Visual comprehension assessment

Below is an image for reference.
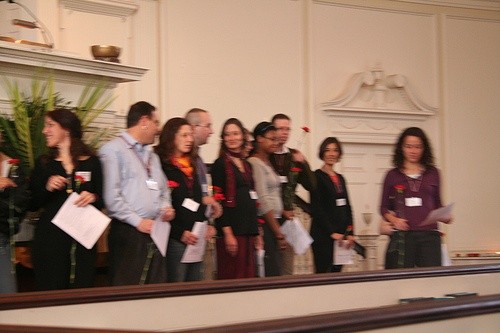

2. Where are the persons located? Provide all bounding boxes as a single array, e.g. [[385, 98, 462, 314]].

[[211, 118, 260, 280], [227, 128, 265, 276], [308, 136, 356, 274], [22, 109, 103, 287], [380, 128, 454, 269], [246, 120, 293, 277], [0, 127, 39, 294], [265, 113, 317, 275], [153, 118, 206, 283], [184, 108, 223, 225], [94, 101, 176, 284]]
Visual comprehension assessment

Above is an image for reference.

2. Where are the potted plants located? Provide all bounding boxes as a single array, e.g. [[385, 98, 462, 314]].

[[0, 71, 122, 293]]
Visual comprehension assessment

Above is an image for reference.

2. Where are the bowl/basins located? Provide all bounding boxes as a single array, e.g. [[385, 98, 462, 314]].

[[91, 45, 120, 63]]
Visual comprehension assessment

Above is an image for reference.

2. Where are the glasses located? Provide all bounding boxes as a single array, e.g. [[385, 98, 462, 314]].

[[261, 134, 278, 141], [195, 123, 211, 128]]
